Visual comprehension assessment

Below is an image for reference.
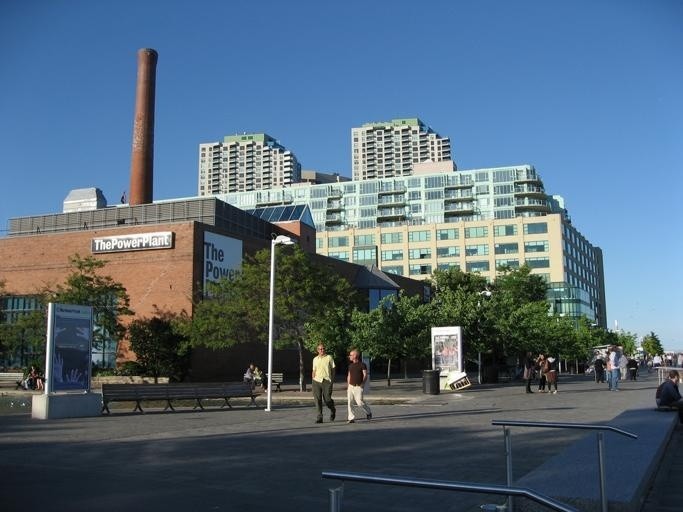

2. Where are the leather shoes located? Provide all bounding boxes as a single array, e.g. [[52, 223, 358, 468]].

[[314, 420, 322, 424], [329, 413, 334, 421]]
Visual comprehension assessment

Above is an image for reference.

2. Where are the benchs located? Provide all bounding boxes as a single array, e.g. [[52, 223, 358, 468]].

[[101, 381, 262, 415], [265, 373, 284, 392]]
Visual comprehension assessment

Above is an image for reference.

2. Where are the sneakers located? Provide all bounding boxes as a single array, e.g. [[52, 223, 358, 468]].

[[526, 390, 557, 394], [346, 420, 354, 423], [366, 413, 370, 420]]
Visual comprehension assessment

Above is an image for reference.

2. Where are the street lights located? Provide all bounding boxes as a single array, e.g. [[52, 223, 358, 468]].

[[477, 286, 491, 384], [264, 232, 295, 412]]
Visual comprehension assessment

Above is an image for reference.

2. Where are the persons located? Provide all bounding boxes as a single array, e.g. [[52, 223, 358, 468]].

[[244, 362, 256, 385], [543, 353, 560, 395], [656, 370, 682, 424], [32, 369, 36, 379], [347, 349, 372, 424], [23, 365, 38, 390], [254, 364, 267, 394], [594, 346, 683, 395], [522, 351, 537, 394], [536, 354, 547, 394], [310, 344, 338, 423], [35, 367, 45, 392]]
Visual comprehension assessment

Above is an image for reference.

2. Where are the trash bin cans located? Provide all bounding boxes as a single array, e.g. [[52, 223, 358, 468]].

[[422, 369, 441, 395]]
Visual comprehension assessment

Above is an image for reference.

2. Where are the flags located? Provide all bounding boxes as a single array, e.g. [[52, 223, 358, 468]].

[[120, 192, 126, 205]]
